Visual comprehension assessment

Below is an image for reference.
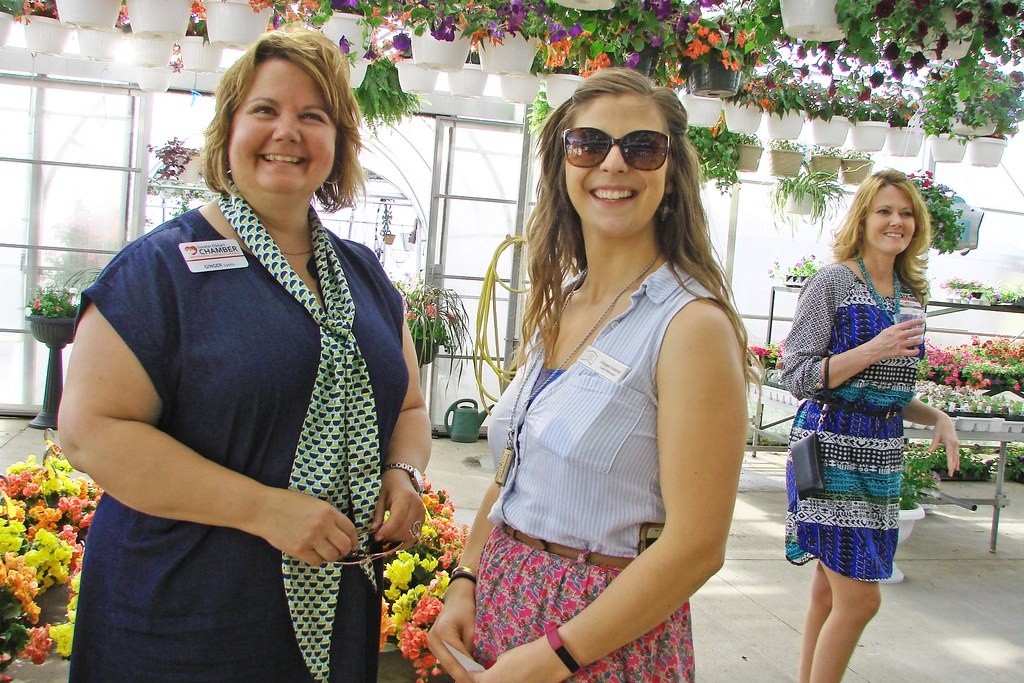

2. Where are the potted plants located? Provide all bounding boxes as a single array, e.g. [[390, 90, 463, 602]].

[[380, 202, 396, 246], [767, 170, 853, 244]]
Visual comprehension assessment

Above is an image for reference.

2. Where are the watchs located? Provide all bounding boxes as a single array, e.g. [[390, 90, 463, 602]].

[[381, 462, 424, 492]]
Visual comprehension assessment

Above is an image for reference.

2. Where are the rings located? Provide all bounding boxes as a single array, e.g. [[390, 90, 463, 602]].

[[409, 520, 424, 539]]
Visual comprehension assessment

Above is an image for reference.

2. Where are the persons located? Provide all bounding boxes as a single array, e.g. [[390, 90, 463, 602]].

[[58, 28, 434, 683], [782, 168, 960, 683], [426, 67, 766, 683]]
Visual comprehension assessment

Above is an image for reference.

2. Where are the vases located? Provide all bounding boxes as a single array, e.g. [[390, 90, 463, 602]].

[[21, 314, 81, 430], [749, 357, 1024, 432], [414, 334, 443, 363], [0, 0, 274, 95], [786, 275, 1024, 306], [879, 465, 1024, 584], [320, 0, 1024, 249]]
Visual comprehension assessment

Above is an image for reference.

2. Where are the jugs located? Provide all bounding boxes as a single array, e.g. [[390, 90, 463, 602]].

[[444, 398, 494, 442]]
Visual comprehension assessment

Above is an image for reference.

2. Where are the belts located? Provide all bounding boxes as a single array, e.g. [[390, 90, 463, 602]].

[[501, 522, 634, 570]]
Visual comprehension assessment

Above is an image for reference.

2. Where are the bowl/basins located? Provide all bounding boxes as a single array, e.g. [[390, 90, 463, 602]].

[[391, 249, 409, 263]]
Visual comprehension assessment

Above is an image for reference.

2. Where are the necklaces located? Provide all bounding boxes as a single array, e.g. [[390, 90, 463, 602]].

[[494, 249, 663, 488], [853, 253, 901, 322], [283, 250, 313, 255]]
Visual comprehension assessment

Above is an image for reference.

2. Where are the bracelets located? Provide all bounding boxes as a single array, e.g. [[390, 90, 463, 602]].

[[545, 623, 583, 678], [452, 566, 477, 576], [447, 573, 478, 586]]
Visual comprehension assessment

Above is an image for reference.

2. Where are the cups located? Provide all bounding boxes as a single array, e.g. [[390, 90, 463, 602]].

[[893, 313, 926, 344], [400, 233, 414, 251]]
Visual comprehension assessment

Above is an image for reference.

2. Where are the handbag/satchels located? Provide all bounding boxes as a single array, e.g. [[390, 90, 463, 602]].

[[792, 357, 837, 502]]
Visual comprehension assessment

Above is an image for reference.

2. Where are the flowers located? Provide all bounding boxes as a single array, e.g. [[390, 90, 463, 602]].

[[906, 169, 966, 257], [14, 0, 1024, 160], [0, 445, 471, 683], [749, 337, 1023, 414], [28, 285, 82, 319], [767, 257, 1020, 296], [898, 442, 1024, 510], [394, 281, 473, 345]]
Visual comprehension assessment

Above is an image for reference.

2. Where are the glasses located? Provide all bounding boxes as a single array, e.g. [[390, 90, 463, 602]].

[[334, 528, 404, 565], [560, 126, 674, 171]]
